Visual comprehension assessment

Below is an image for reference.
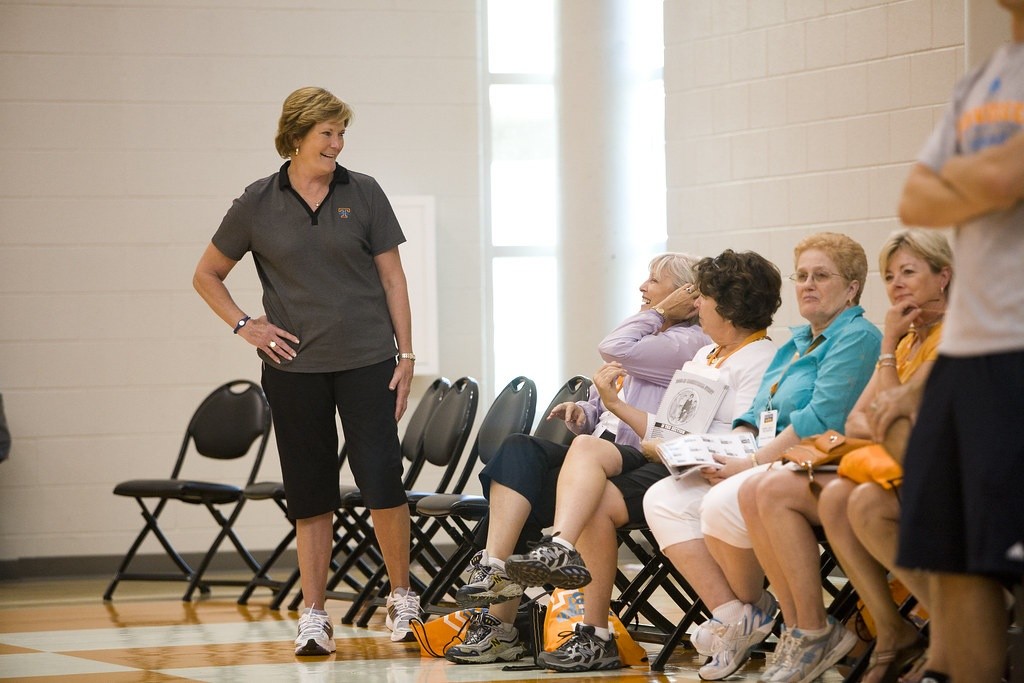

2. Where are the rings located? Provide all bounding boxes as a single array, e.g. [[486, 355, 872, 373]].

[[269, 342, 276, 348], [686, 288, 693, 294], [871, 402, 877, 409]]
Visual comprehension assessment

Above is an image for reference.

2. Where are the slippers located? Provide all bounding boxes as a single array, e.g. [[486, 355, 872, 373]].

[[861, 632, 928, 682]]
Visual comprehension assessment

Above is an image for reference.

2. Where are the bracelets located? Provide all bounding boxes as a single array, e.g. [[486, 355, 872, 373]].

[[877, 354, 897, 363], [750, 454, 758, 467], [397, 353, 416, 361], [878, 363, 897, 370]]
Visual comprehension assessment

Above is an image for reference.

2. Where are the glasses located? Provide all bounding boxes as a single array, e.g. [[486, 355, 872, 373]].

[[789, 269, 853, 286], [903, 298, 945, 333]]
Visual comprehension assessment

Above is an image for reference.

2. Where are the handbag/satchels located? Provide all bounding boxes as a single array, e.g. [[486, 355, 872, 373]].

[[767, 428, 873, 482], [837, 443, 907, 491], [409, 607, 490, 658], [542, 583, 651, 666]]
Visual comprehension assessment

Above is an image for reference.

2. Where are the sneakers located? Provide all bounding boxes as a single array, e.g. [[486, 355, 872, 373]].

[[536, 623, 621, 672], [698, 603, 777, 681], [445, 611, 528, 664], [455, 548, 524, 610], [385, 586, 425, 642], [690, 590, 778, 657], [756, 613, 858, 683], [295, 602, 337, 656], [504, 532, 593, 590]]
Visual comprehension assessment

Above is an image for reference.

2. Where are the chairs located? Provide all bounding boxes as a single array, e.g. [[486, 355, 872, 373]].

[[102, 378, 1024, 683]]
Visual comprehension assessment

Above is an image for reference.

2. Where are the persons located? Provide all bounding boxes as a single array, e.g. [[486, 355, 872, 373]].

[[193, 87, 425, 656], [894, 0, 1024, 683], [739, 228, 958, 683], [505, 248, 782, 671], [444, 252, 713, 665], [643, 233, 883, 680]]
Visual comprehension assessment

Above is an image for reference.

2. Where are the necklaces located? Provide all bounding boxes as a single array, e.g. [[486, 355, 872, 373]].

[[290, 173, 330, 207], [912, 314, 944, 331]]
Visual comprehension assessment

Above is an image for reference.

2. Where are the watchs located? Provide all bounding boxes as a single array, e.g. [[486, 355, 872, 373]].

[[233, 316, 251, 334], [651, 306, 668, 323]]
